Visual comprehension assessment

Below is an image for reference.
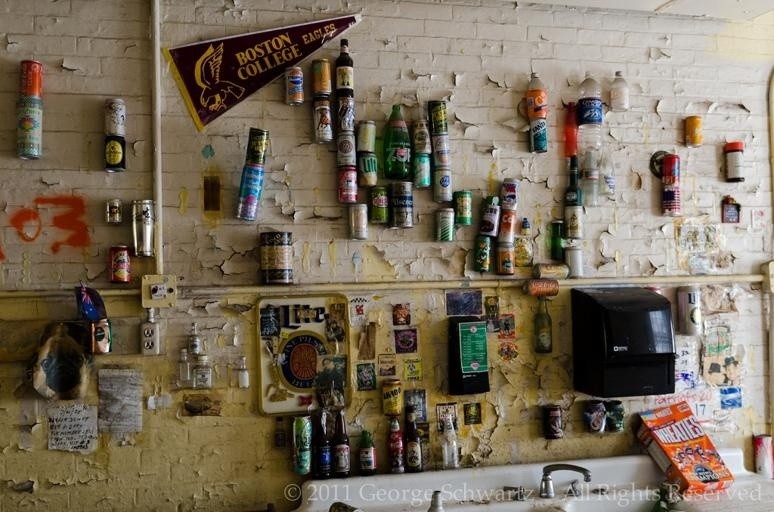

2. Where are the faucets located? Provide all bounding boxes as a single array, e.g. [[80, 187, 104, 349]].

[[539, 462, 594, 499]]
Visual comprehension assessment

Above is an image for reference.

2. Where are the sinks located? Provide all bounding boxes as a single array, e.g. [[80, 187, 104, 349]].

[[293, 448, 774, 511]]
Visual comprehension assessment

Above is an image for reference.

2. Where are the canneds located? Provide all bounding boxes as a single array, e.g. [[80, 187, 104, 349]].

[[530, 118, 547, 154], [91, 317, 112, 355], [677, 285, 701, 337], [101, 97, 126, 173], [290, 414, 313, 475], [281, 58, 518, 275], [105, 197, 156, 281], [522, 263, 571, 297], [381, 378, 402, 416], [234, 127, 294, 284], [14, 60, 43, 160], [754, 433, 773, 480], [542, 400, 625, 439], [660, 114, 704, 217]]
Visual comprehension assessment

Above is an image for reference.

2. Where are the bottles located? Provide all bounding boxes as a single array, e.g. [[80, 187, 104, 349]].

[[385, 416, 403, 473], [404, 403, 422, 470], [582, 149, 600, 207], [577, 70, 601, 150], [550, 221, 564, 258], [180, 323, 249, 389], [272, 415, 285, 448], [610, 71, 629, 113], [358, 431, 375, 475], [535, 296, 553, 352], [335, 35, 353, 96], [564, 156, 583, 238], [563, 102, 578, 157], [329, 408, 351, 477], [441, 414, 460, 467], [724, 142, 745, 182], [312, 410, 330, 480], [385, 102, 412, 177], [527, 71, 546, 119]]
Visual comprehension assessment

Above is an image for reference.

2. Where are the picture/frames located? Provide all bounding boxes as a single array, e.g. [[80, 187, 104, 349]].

[[256, 294, 352, 416]]
